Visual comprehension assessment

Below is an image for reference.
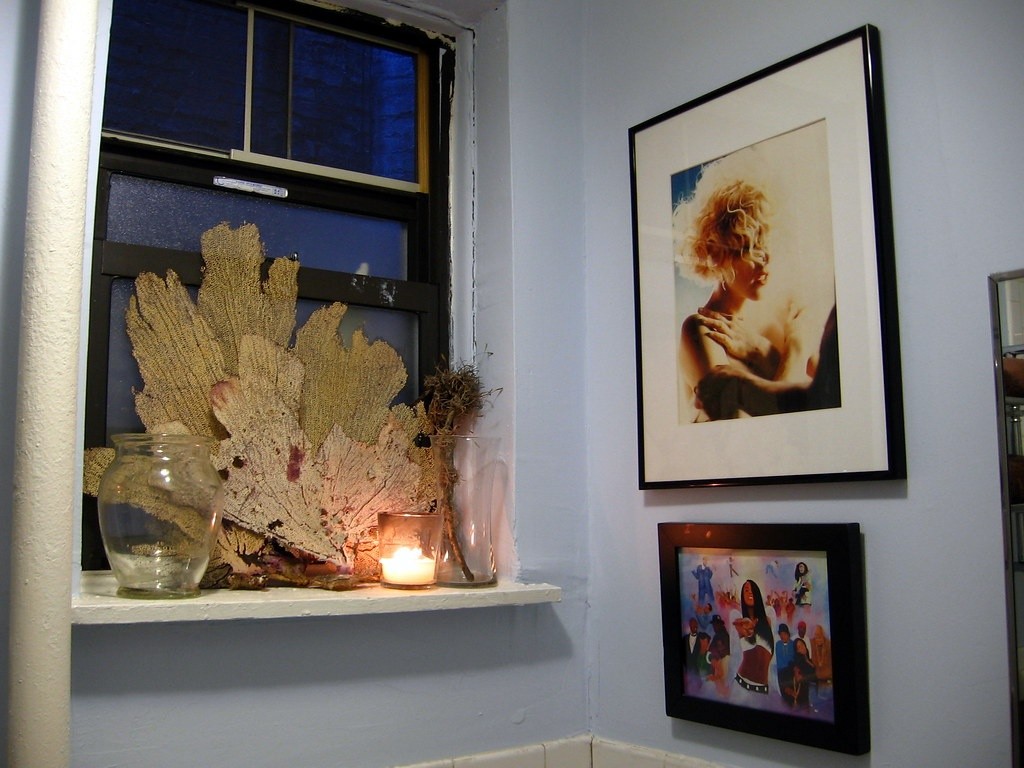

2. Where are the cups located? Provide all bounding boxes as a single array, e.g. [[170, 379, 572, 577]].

[[98, 433, 224, 598]]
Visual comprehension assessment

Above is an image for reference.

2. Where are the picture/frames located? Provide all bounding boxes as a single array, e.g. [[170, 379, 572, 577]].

[[655, 521, 871, 755], [626, 24, 908, 491]]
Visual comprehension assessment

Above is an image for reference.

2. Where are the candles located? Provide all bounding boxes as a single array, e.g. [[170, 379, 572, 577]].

[[377, 546, 434, 585]]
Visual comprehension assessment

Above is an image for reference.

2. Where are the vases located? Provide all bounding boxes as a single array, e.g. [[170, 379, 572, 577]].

[[430, 436, 498, 588], [97, 433, 224, 599]]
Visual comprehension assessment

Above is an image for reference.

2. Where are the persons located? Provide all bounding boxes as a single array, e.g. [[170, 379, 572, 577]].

[[677, 158, 842, 424]]
